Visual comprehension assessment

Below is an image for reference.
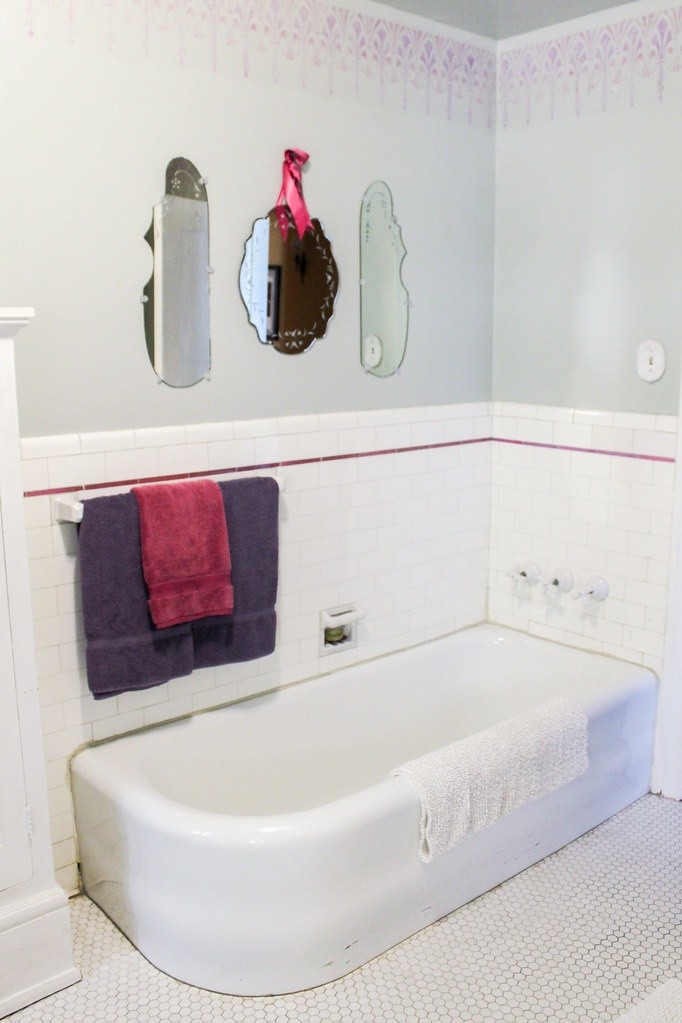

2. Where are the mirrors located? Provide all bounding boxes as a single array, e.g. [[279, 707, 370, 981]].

[[358, 179, 409, 378], [143, 157, 211, 387], [239, 204, 339, 354]]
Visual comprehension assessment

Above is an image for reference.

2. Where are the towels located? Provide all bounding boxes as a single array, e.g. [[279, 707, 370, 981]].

[[384, 697, 589, 863], [131, 480, 233, 629], [78, 477, 279, 700]]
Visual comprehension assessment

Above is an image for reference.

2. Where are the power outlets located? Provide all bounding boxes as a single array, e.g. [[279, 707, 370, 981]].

[[362, 335, 384, 368], [636, 340, 665, 383]]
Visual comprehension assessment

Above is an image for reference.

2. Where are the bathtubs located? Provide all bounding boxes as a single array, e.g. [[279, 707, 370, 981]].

[[71, 621, 660, 997]]
